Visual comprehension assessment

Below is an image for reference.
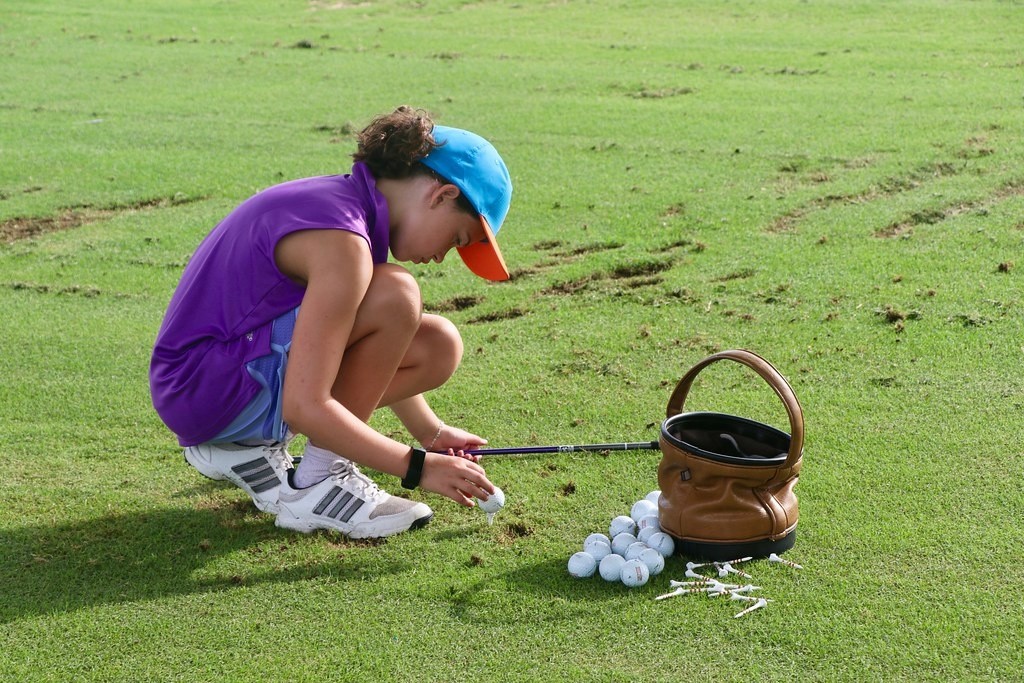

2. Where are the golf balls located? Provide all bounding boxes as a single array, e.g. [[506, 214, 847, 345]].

[[476, 485, 506, 514], [567, 489, 675, 589]]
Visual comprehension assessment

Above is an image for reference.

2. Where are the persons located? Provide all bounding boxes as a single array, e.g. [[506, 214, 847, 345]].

[[147, 106, 510, 539]]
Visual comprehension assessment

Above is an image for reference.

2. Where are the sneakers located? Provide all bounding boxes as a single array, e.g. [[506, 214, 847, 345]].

[[273, 461, 434, 538], [185, 439, 295, 516]]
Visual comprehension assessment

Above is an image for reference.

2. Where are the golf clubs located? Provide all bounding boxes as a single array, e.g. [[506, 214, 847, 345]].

[[416, 440, 662, 458]]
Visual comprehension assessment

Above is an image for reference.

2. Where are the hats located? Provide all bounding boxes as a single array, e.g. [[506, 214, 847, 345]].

[[418, 124, 512, 282]]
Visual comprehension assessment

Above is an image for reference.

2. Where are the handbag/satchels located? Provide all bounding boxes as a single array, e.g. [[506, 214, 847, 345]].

[[657, 349, 806, 560]]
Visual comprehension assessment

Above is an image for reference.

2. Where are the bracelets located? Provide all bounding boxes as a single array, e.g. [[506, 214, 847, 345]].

[[428, 421, 444, 451], [401, 447, 426, 490]]
